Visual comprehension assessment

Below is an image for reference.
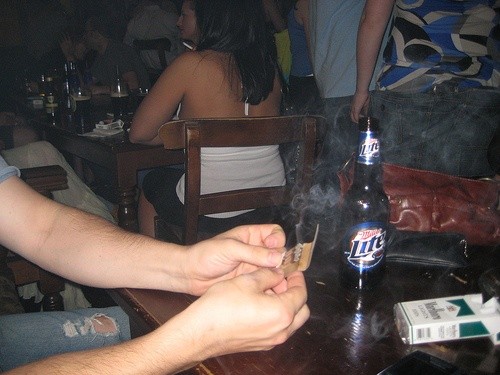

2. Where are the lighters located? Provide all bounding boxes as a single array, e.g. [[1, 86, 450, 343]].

[[99, 118, 114, 125]]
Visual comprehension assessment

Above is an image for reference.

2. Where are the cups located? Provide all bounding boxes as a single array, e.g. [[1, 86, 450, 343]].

[[110, 80, 129, 116], [136, 87, 150, 105], [73, 87, 91, 112]]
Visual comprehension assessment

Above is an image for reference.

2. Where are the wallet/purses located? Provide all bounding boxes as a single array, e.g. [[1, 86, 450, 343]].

[[385, 223, 469, 268]]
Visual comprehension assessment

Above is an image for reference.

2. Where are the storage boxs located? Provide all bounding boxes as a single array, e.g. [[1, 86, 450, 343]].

[[393, 293, 500, 348], [95, 118, 125, 130]]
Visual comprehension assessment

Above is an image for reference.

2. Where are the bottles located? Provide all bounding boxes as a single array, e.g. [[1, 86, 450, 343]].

[[39, 61, 81, 119], [337, 117, 390, 293]]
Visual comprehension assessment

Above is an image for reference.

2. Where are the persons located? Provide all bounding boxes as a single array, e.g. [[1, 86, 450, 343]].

[[0, 139, 120, 315], [0, 155, 311, 375], [0, 0, 500, 240]]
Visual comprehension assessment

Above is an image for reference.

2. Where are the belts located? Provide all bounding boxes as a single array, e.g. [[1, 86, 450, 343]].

[[425, 84, 465, 99]]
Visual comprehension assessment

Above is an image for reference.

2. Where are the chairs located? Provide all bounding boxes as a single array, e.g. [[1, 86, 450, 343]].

[[0, 38, 333, 311]]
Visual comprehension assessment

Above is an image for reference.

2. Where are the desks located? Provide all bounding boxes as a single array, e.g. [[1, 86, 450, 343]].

[[122, 220, 500, 375], [29, 109, 182, 228]]
